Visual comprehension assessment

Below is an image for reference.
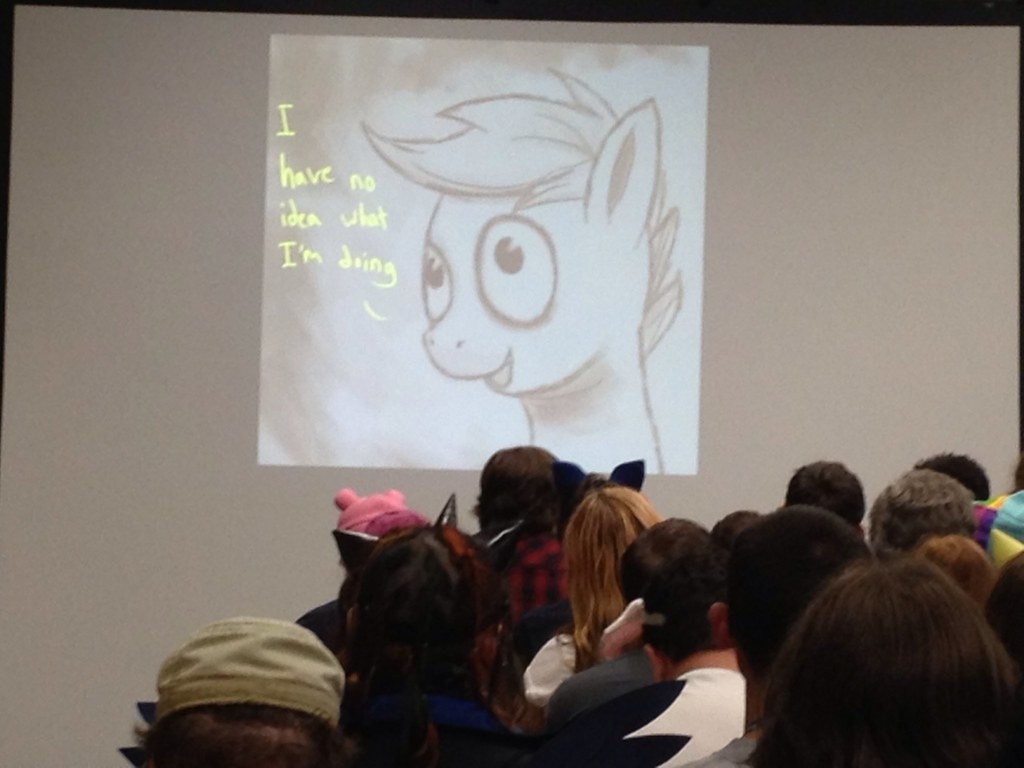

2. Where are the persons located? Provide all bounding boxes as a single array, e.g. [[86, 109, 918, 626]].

[[116, 444, 1024, 768]]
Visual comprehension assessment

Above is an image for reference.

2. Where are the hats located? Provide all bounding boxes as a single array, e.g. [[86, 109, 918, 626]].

[[333, 486, 430, 541], [153, 618, 346, 731]]
